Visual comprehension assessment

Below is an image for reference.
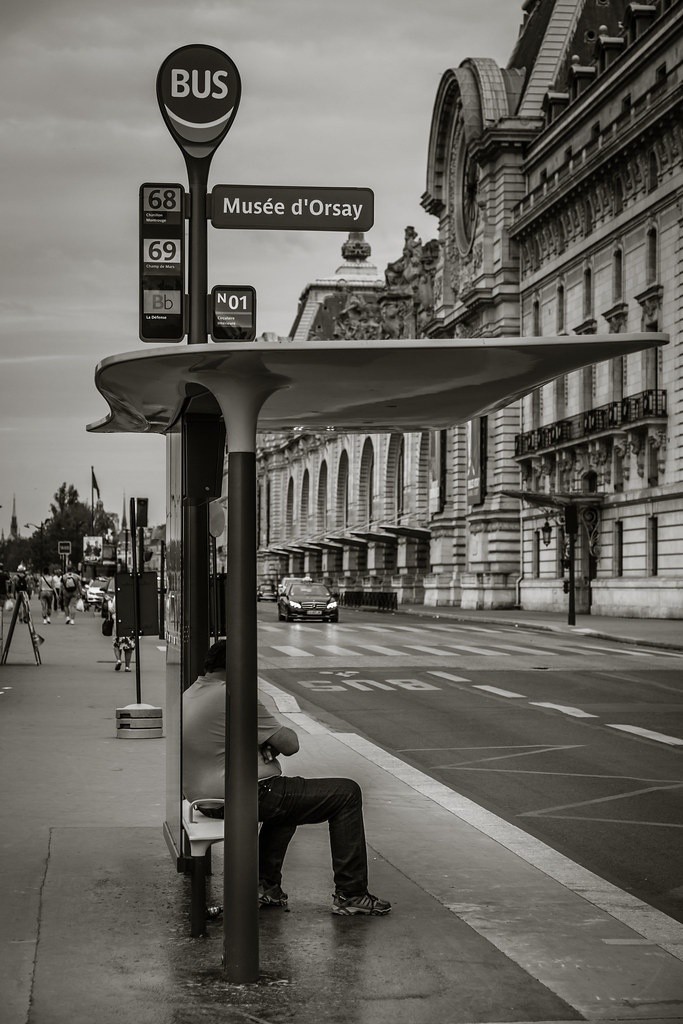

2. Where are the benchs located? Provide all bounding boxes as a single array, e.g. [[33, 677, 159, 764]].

[[182, 797, 261, 856]]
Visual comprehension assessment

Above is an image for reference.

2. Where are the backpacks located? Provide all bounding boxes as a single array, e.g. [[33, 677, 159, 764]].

[[65, 573, 76, 592], [16, 573, 28, 592]]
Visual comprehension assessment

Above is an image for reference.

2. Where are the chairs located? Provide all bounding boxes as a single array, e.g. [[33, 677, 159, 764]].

[[293, 588, 302, 595], [312, 589, 320, 595]]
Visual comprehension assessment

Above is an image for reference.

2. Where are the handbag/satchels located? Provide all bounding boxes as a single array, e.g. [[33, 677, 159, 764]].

[[102, 618, 114, 636], [76, 598, 85, 612], [6, 598, 13, 611]]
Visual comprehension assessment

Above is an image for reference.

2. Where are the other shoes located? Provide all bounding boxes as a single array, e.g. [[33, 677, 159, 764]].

[[24, 618, 27, 622], [48, 617, 51, 624], [44, 619, 47, 625], [66, 618, 75, 625], [125, 667, 131, 672], [115, 660, 122, 670]]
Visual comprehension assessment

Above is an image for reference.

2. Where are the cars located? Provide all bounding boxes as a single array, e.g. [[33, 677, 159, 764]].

[[257, 583, 278, 602], [81, 577, 115, 618], [277, 577, 340, 623]]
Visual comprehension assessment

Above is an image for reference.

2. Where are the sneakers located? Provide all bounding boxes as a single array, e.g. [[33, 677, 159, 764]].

[[331, 888, 392, 916], [258, 884, 288, 904]]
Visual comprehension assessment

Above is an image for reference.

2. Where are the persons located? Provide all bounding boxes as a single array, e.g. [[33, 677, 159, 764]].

[[38, 564, 86, 627], [106, 566, 136, 672], [0, 565, 37, 623], [182, 639, 392, 916]]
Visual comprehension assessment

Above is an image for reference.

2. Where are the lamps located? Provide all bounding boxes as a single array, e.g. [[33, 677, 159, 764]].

[[542, 512, 565, 547]]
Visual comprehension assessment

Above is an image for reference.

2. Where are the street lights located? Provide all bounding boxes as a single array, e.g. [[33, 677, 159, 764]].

[[23, 521, 44, 600], [541, 496, 579, 625]]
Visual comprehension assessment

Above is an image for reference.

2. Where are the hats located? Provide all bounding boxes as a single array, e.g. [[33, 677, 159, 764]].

[[17, 565, 26, 572]]
